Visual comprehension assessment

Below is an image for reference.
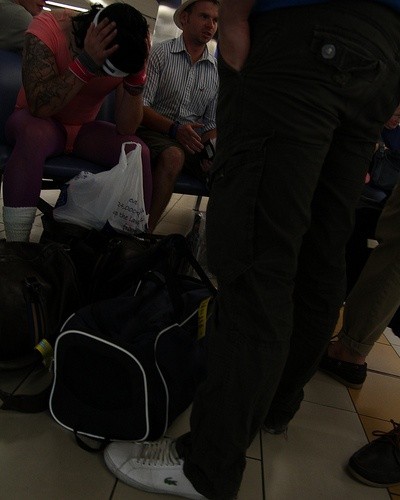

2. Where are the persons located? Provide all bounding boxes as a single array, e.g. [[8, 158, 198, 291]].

[[104, 0, 400, 500], [3, 2, 152, 243], [141, 0, 220, 234], [318, 171, 400, 487], [344, 105, 400, 296], [0, 0, 46, 52]]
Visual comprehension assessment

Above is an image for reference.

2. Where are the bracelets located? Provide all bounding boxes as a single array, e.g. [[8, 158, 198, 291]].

[[123, 63, 148, 96], [67, 49, 100, 84], [168, 121, 180, 138]]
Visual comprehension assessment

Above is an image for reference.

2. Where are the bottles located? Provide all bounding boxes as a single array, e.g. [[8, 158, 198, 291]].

[[181, 213, 202, 277]]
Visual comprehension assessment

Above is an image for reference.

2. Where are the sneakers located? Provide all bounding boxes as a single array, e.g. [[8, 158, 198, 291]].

[[347, 419, 400, 488], [103, 437, 210, 500]]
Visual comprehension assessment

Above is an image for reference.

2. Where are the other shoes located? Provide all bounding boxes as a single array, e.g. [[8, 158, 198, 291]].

[[318, 354, 367, 390]]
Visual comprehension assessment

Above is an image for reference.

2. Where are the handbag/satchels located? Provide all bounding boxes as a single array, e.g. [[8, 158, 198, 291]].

[[0, 220, 223, 453]]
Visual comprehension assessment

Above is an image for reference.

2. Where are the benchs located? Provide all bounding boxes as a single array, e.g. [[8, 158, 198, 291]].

[[0, 49, 211, 211]]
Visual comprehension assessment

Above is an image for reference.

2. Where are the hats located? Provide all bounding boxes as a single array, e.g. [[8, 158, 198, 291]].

[[173, 0, 224, 31]]
